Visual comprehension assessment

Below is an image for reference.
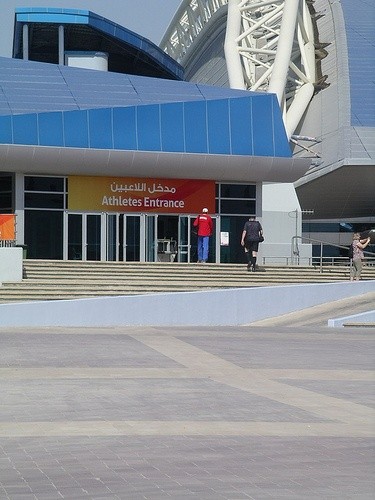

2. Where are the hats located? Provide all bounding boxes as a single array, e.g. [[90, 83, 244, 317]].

[[202, 208, 208, 214]]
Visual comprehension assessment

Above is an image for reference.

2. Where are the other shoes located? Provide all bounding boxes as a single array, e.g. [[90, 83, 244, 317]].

[[252, 268, 255, 271], [197, 260, 207, 263], [247, 262, 252, 268]]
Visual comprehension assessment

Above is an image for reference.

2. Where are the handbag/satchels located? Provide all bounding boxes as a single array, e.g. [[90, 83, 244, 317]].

[[192, 215, 201, 239], [258, 225, 264, 242], [347, 244, 353, 258]]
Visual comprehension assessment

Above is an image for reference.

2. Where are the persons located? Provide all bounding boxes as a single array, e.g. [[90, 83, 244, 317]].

[[240, 218, 264, 272], [350, 232, 370, 283], [194, 207, 214, 263]]
